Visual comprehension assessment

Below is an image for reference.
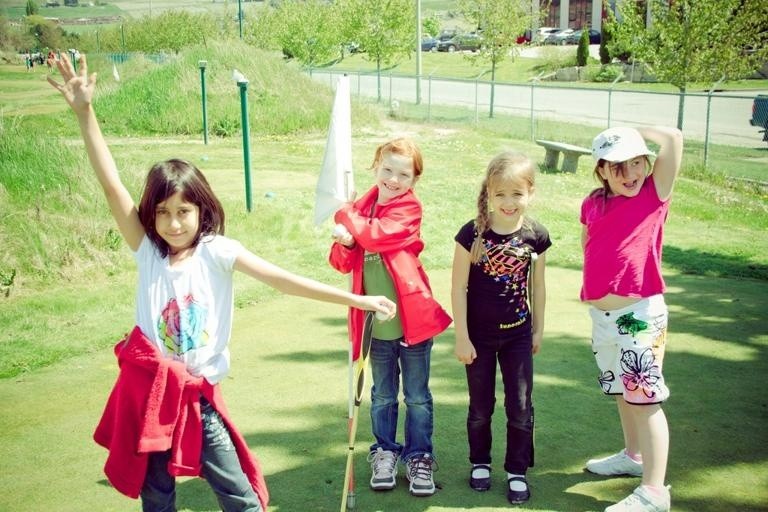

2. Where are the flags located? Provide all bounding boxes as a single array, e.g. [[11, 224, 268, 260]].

[[314, 72, 357, 231]]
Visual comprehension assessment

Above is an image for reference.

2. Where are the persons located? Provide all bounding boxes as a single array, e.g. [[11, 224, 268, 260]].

[[328, 137, 454, 496], [450, 150, 554, 506], [577, 122, 684, 512], [47, 50, 399, 510], [23, 48, 56, 73]]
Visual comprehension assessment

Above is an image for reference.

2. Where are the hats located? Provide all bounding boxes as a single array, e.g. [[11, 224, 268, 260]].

[[590, 125, 657, 165]]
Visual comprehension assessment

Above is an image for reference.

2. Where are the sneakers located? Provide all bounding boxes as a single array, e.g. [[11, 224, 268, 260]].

[[404, 453, 435, 495], [603, 483, 671, 511], [585, 448, 647, 478], [508, 475, 530, 505], [369, 447, 400, 490], [468, 466, 492, 491]]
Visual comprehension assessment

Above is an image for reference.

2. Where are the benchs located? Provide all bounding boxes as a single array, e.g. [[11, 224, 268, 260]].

[[535, 139, 592, 174]]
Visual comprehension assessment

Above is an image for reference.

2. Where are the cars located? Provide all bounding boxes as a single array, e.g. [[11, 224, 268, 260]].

[[749, 94, 768, 141], [421, 25, 600, 53]]
